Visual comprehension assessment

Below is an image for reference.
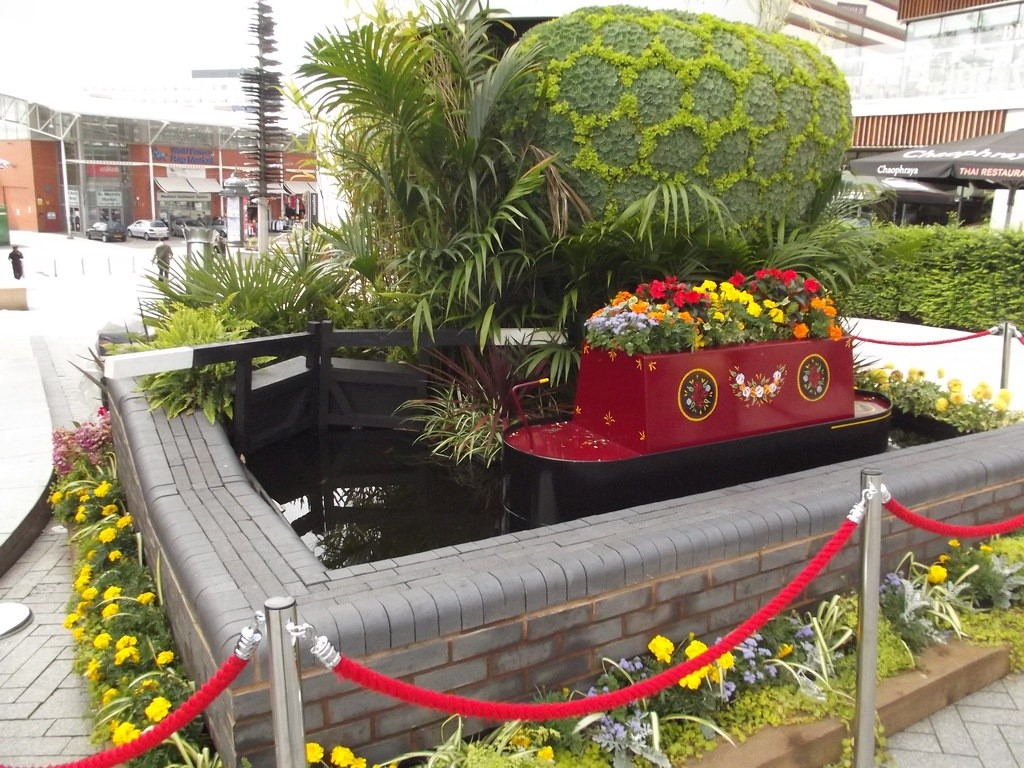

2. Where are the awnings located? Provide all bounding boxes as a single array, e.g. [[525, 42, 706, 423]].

[[284, 180, 317, 194], [155, 176, 223, 194]]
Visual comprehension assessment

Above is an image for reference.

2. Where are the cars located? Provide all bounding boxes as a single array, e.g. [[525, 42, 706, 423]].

[[200, 219, 228, 239], [86, 220, 127, 242], [127, 219, 171, 241], [169, 217, 209, 238]]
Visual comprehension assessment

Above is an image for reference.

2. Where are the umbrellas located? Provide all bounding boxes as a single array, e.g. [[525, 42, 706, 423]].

[[843, 168, 954, 204], [853, 127, 1024, 231]]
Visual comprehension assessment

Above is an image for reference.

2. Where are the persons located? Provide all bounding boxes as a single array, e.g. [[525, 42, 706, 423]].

[[8, 246, 23, 279], [152, 238, 174, 282]]
